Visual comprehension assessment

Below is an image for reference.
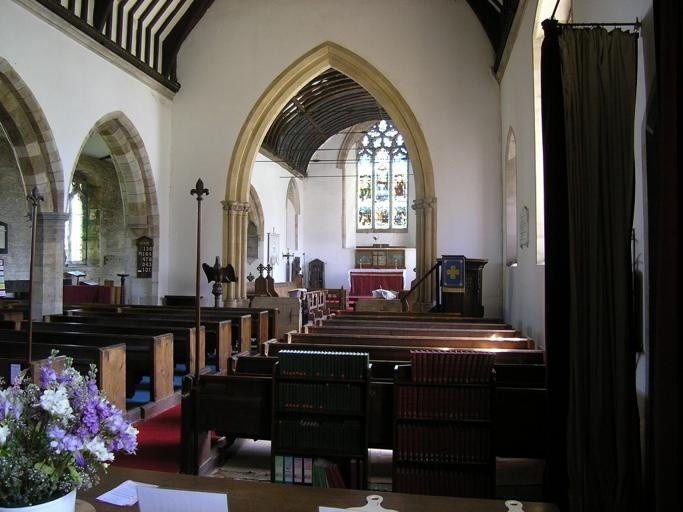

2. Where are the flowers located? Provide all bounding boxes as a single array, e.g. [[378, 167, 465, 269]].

[[0, 349, 140, 508]]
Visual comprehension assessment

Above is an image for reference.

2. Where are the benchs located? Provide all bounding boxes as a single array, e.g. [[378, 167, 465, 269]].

[[0, 303, 551, 476]]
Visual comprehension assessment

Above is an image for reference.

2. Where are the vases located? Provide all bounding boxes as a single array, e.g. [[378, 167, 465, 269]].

[[0, 485, 77, 512]]
